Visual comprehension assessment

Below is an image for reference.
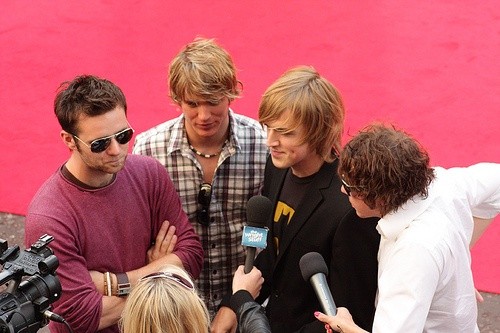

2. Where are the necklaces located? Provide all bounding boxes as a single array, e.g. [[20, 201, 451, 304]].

[[187, 135, 230, 158]]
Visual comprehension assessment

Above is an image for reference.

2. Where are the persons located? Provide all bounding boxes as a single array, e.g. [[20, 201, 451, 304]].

[[314, 305, 369, 333], [337, 122, 500, 333], [207, 65, 380, 333], [119, 264, 271, 333], [25, 75, 206, 333], [133, 39, 270, 322]]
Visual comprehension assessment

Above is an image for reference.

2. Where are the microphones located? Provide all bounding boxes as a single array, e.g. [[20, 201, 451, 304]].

[[241, 195, 272, 274], [300, 252, 341, 333]]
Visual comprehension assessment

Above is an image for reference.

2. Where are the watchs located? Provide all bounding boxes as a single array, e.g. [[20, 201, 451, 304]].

[[116, 272, 130, 297]]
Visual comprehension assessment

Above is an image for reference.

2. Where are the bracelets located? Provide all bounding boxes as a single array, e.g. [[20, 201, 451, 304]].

[[104, 271, 114, 296]]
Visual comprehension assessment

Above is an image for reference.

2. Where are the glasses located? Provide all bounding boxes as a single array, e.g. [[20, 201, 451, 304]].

[[139, 270, 194, 289], [73, 119, 135, 152], [342, 179, 364, 196], [195, 182, 212, 226]]
